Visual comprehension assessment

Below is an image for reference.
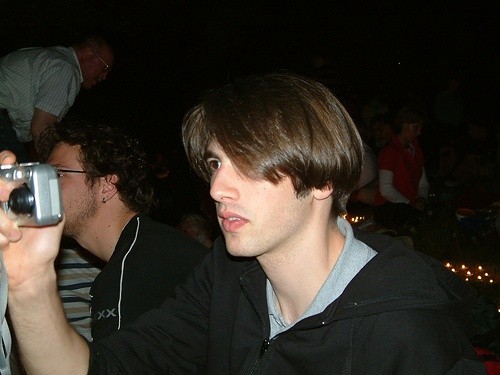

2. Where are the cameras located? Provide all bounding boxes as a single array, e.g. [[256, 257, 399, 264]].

[[0, 162, 62, 227]]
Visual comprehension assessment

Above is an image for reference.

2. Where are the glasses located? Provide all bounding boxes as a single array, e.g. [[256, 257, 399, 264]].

[[91, 49, 110, 71]]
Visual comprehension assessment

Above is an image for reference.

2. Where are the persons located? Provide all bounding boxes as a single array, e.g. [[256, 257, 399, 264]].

[[0, 70, 487, 375], [0, 35, 211, 375], [177, 54, 500, 256]]
